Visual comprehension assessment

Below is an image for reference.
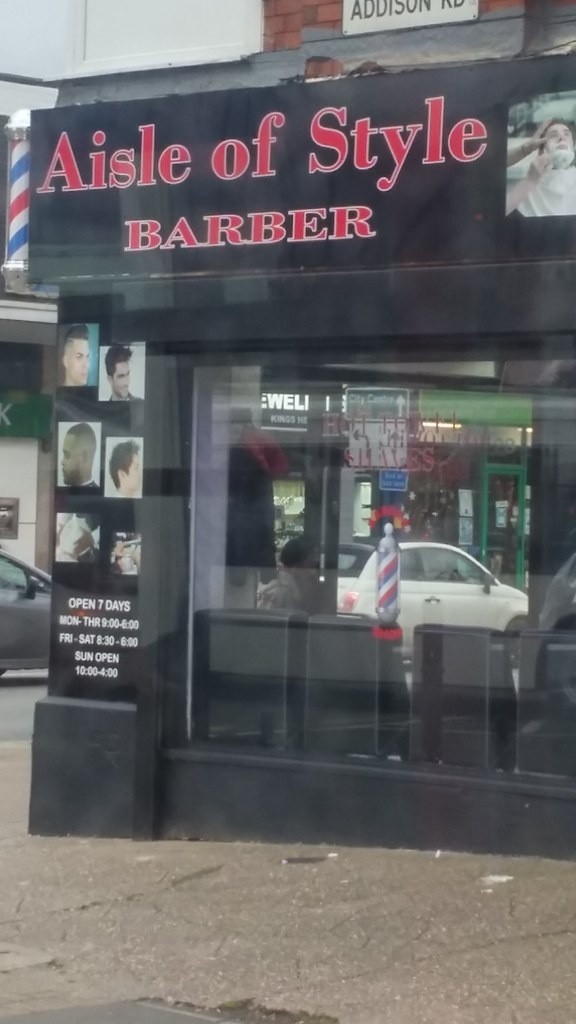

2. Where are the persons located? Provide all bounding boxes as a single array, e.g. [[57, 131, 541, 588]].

[[506, 118, 576, 217], [61, 323, 90, 386], [104, 344, 143, 402], [108, 438, 141, 498], [60, 424, 99, 487], [256, 535, 327, 612]]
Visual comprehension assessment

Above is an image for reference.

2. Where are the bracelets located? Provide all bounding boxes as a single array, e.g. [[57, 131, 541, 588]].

[[519, 142, 527, 157]]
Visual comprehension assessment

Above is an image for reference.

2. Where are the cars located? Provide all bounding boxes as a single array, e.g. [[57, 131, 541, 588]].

[[0, 548, 52, 684], [320, 540, 530, 661], [535, 551, 575, 722]]
